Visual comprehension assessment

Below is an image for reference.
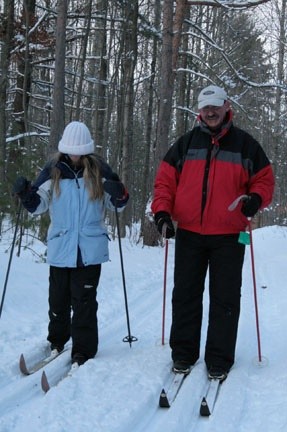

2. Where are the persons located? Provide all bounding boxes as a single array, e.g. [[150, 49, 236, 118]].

[[12, 121, 130, 366], [151, 85, 275, 378]]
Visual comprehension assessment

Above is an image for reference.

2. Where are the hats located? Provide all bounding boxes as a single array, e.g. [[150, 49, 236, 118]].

[[196, 84, 229, 110], [57, 121, 94, 156]]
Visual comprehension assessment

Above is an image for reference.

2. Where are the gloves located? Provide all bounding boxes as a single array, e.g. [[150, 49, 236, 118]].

[[239, 193, 262, 217], [154, 210, 175, 238], [12, 177, 38, 209], [102, 172, 125, 200]]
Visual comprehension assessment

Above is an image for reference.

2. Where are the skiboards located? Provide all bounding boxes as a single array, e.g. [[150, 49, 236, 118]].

[[19, 341, 73, 392], [159, 372, 220, 416]]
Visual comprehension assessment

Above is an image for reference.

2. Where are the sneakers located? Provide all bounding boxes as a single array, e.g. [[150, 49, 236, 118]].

[[208, 365, 226, 378], [71, 352, 87, 365], [51, 343, 65, 352], [173, 359, 190, 373]]
